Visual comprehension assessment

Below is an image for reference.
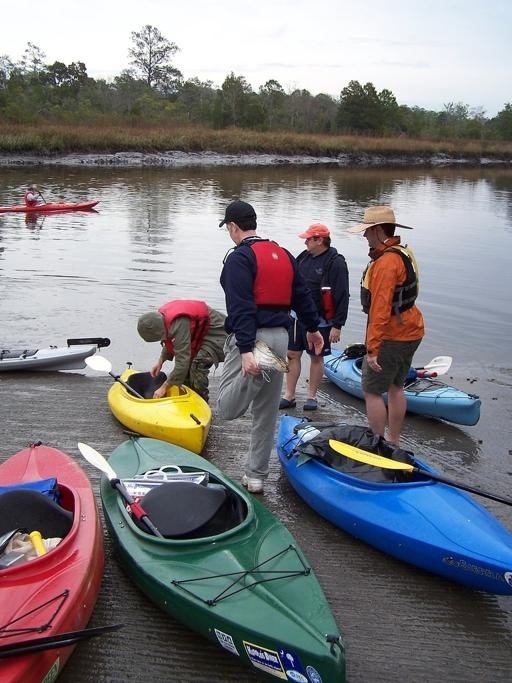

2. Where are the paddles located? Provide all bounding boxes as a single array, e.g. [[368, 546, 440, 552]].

[[84, 355, 144, 400], [0, 622, 126, 659], [77, 441, 165, 539], [415, 356, 453, 375], [328, 438, 511, 507]]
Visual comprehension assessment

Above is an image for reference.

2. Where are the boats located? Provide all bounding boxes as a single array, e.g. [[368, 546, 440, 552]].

[[0, 445, 105, 683], [0, 199, 99, 214], [277, 412, 512, 596], [100, 436, 346, 682], [107, 369, 212, 455], [0, 343, 100, 371], [323, 343, 482, 426]]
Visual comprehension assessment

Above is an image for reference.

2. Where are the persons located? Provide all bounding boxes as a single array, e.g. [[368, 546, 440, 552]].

[[136, 299, 230, 403], [24, 187, 41, 207], [23, 212, 46, 241], [277, 223, 350, 412], [216, 199, 323, 495], [345, 203, 425, 447]]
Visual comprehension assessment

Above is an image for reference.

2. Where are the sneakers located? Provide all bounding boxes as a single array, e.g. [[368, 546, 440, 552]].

[[252, 340, 289, 374], [241, 475, 265, 492]]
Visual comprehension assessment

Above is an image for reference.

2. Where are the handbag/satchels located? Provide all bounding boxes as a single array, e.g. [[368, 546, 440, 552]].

[[119, 464, 210, 503]]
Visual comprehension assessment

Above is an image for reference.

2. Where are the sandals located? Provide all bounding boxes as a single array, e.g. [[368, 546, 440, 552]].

[[278, 398, 297, 409], [304, 397, 318, 409]]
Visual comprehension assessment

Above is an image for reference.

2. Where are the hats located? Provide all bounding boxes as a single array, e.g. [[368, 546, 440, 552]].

[[298, 224, 330, 238], [346, 207, 415, 232], [218, 201, 256, 227]]
